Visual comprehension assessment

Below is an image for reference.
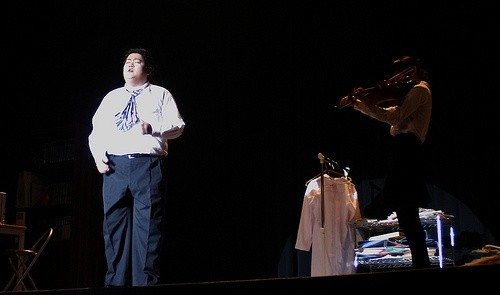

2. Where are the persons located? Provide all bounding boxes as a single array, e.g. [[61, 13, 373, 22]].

[[86, 48, 186, 287], [338, 55, 433, 271]]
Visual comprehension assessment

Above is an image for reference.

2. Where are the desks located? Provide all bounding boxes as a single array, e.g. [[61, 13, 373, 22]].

[[0, 223, 27, 291]]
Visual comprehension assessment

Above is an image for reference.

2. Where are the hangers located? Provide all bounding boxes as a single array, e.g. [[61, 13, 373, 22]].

[[304, 157, 356, 189]]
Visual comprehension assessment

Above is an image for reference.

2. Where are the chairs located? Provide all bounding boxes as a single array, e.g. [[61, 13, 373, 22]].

[[2, 228, 54, 292]]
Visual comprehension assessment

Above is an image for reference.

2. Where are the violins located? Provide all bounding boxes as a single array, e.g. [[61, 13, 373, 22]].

[[339, 65, 417, 109]]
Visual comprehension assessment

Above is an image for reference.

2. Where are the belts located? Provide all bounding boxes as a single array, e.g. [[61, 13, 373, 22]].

[[111, 154, 159, 159]]
